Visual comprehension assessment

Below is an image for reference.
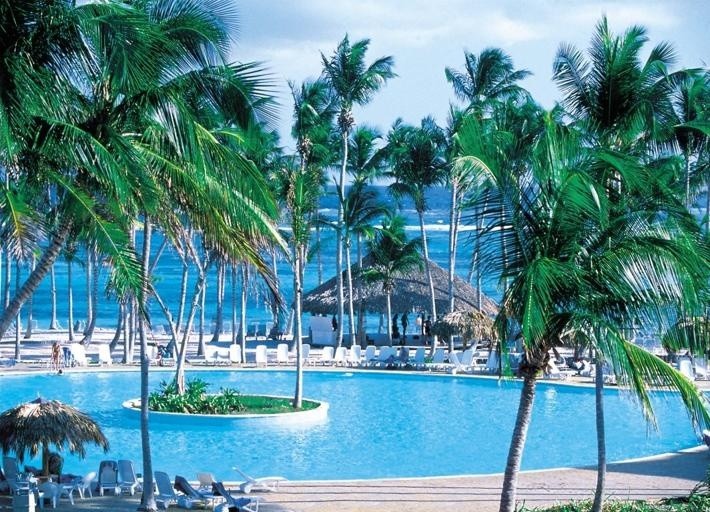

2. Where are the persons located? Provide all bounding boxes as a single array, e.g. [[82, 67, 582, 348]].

[[332, 315, 338, 331], [155, 342, 170, 359], [51, 342, 60, 371], [154, 351, 162, 366], [57, 370, 64, 376], [391, 311, 447, 351]]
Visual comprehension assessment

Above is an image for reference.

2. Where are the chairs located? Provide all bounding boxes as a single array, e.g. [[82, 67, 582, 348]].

[[427, 347, 444, 370], [256, 345, 268, 367], [334, 347, 347, 366], [0, 456, 288, 511], [303, 344, 317, 364], [347, 344, 362, 367], [318, 346, 333, 364], [70, 343, 92, 367], [278, 343, 289, 366], [98, 343, 114, 365], [146, 345, 176, 367], [361, 346, 378, 368], [446, 340, 579, 381], [204, 345, 218, 364], [231, 343, 242, 364], [373, 345, 410, 367], [407, 347, 427, 369]]
